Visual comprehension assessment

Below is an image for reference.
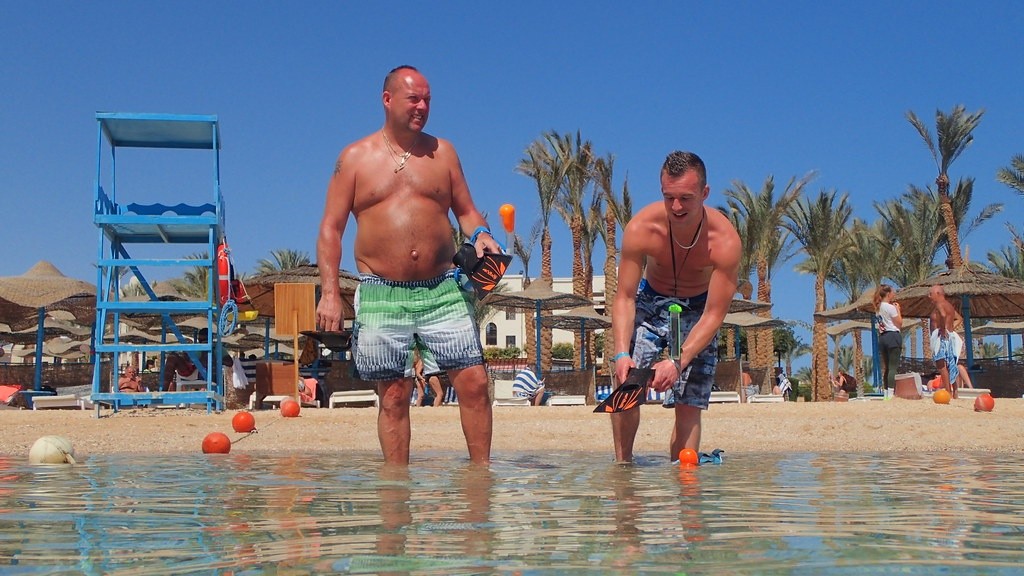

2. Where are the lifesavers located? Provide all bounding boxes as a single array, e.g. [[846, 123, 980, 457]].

[[218, 244, 229, 306]]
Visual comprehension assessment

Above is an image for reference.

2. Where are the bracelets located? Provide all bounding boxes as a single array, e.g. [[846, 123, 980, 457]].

[[670, 359, 682, 374], [614, 352, 630, 361], [470, 226, 491, 245]]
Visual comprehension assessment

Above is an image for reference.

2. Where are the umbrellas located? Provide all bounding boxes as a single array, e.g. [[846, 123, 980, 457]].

[[0, 261, 359, 390], [486, 259, 1024, 381]]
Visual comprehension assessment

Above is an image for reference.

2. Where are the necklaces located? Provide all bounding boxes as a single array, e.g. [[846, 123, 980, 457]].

[[381, 129, 416, 173], [672, 221, 702, 249]]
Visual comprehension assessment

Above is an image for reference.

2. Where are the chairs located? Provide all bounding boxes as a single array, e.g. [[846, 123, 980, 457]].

[[541, 392, 586, 406], [709, 392, 741, 403], [493, 379, 531, 406], [746, 395, 784, 403], [32, 384, 105, 410], [249, 378, 321, 409], [328, 389, 379, 408]]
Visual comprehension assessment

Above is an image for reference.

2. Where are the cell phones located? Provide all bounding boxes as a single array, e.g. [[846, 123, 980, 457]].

[[671, 317, 679, 358]]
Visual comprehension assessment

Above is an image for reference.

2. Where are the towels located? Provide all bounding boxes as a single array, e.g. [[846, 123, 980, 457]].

[[231, 357, 250, 390], [512, 366, 545, 395], [931, 329, 960, 385], [299, 377, 319, 403]]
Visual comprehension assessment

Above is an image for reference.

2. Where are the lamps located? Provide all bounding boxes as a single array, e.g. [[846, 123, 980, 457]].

[[922, 385, 991, 399]]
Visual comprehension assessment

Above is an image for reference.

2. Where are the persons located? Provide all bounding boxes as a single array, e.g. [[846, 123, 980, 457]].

[[834, 370, 848, 392], [736, 365, 752, 403], [315, 65, 503, 463], [119, 327, 312, 396], [874, 283, 903, 399], [413, 347, 444, 406], [609, 150, 743, 461], [928, 284, 972, 399], [512, 363, 544, 406]]
